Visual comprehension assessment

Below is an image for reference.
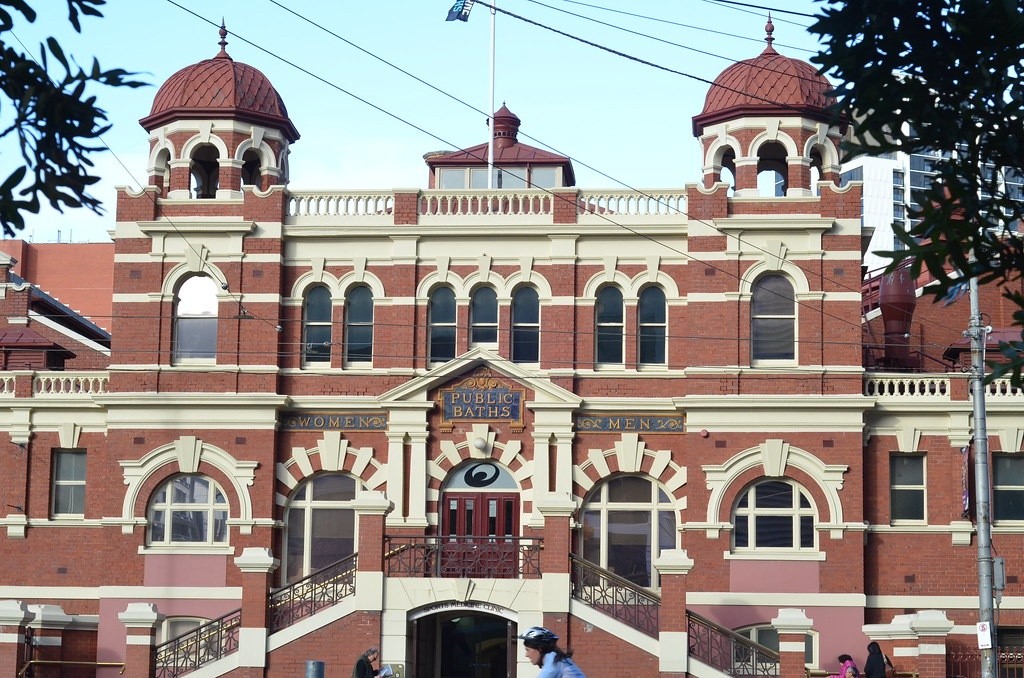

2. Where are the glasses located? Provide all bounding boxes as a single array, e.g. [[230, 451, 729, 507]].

[[372, 655, 376, 660]]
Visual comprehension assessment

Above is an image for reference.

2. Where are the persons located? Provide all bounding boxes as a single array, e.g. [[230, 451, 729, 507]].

[[520, 627, 588, 678], [823, 641, 896, 678], [350, 647, 383, 678]]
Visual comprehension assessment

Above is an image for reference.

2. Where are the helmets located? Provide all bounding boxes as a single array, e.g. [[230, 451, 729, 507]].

[[518, 627, 559, 639]]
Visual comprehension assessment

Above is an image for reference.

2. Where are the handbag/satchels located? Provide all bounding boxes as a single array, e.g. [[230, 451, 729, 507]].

[[882, 654, 898, 678]]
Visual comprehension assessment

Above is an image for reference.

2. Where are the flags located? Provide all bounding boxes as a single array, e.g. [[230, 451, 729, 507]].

[[445, 0, 475, 22]]
